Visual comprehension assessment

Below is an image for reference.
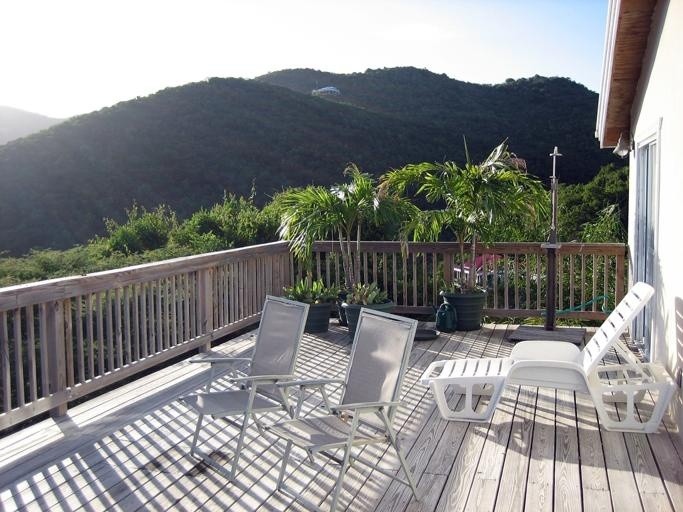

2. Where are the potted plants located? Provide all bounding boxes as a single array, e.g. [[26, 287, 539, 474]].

[[264, 135, 551, 346]]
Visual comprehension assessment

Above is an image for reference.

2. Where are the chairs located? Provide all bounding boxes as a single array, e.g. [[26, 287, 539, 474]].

[[175, 294, 314, 483], [420, 280, 677, 434], [263, 307, 420, 511]]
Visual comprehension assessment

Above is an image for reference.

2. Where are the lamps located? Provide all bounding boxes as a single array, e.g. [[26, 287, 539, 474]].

[[613, 130, 635, 160]]
